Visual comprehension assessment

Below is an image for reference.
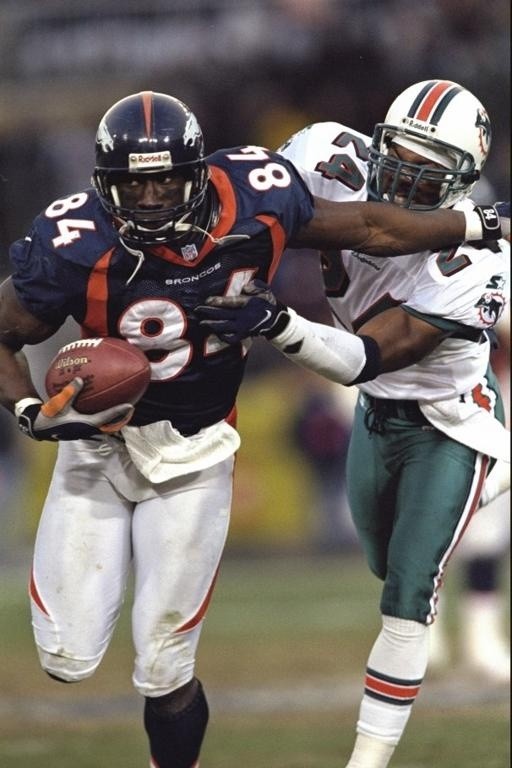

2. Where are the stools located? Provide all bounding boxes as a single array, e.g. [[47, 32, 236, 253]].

[[358, 392, 418, 421]]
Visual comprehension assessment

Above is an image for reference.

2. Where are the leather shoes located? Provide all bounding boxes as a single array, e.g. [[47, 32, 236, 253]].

[[14, 376, 134, 441], [194, 279, 291, 345]]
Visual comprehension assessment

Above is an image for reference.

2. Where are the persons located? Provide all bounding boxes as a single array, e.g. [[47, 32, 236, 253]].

[[290, 241, 511, 686], [1, 93, 509, 763], [193, 81, 511, 768]]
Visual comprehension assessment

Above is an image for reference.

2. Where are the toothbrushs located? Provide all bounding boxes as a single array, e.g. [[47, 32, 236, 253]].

[[45, 338, 151, 426]]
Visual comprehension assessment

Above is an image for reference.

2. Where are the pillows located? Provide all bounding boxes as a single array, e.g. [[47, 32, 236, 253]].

[[376, 80, 492, 203], [91, 91, 206, 210]]
